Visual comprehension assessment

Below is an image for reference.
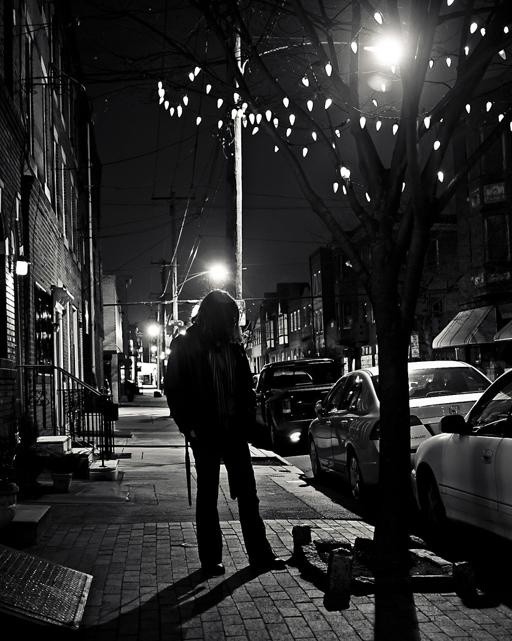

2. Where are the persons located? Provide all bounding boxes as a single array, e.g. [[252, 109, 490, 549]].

[[163, 289, 288, 571]]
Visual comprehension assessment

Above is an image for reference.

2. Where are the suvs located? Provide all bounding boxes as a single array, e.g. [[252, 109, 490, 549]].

[[257, 358, 344, 452]]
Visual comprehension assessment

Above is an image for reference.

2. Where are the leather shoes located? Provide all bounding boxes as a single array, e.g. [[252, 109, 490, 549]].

[[274, 556, 287, 568], [201, 562, 225, 576]]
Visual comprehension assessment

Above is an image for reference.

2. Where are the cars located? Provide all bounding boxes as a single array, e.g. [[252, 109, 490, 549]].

[[413, 370, 511, 537], [310, 362, 512, 502]]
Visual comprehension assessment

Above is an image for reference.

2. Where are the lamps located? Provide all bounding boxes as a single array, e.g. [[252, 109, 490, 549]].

[[15, 252, 32, 278]]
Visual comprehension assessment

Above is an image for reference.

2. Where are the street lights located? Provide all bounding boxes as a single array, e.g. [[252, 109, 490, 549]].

[[168, 266, 228, 332], [230, 31, 409, 323]]
